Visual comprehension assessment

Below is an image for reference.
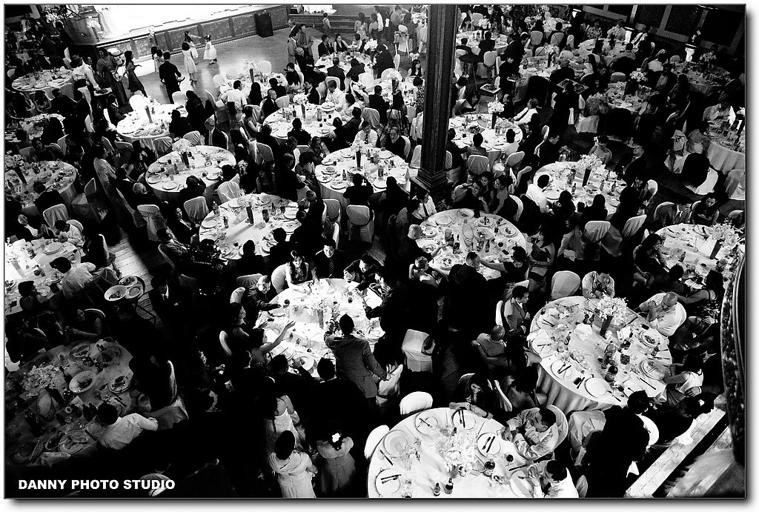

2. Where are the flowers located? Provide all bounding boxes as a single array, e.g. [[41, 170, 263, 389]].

[[350, 141, 369, 157], [171, 138, 191, 156], [629, 67, 648, 81], [699, 51, 717, 63], [609, 27, 620, 37], [4, 155, 22, 170], [706, 221, 737, 242], [440, 431, 477, 472], [293, 94, 310, 106], [735, 107, 745, 119], [238, 193, 255, 209], [575, 153, 600, 173], [6, 251, 17, 263], [487, 100, 505, 115], [309, 292, 330, 311], [597, 296, 627, 321], [21, 365, 55, 398]]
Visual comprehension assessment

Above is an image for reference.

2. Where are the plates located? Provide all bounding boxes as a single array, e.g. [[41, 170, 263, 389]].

[[510, 470, 539, 498], [607, 196, 620, 207], [330, 180, 346, 189], [457, 208, 474, 221], [644, 360, 664, 376], [375, 470, 403, 497], [148, 175, 162, 184], [694, 224, 712, 237], [199, 232, 217, 241], [436, 214, 452, 227], [321, 156, 336, 165], [219, 243, 233, 255], [532, 335, 554, 355], [640, 330, 664, 347], [544, 188, 560, 200], [119, 275, 137, 288], [501, 227, 516, 237], [641, 359, 665, 380], [239, 245, 257, 256], [499, 225, 517, 238], [255, 195, 270, 206], [453, 409, 479, 433], [666, 260, 686, 275], [263, 241, 275, 252], [422, 226, 438, 238], [377, 149, 390, 160], [478, 432, 504, 458], [228, 198, 242, 208], [588, 299, 600, 311], [416, 412, 442, 436], [339, 148, 356, 158], [206, 173, 221, 179], [474, 227, 493, 241], [317, 175, 332, 183], [45, 242, 61, 255], [536, 315, 559, 331], [623, 379, 648, 401], [374, 178, 387, 188], [642, 331, 662, 347], [163, 181, 179, 190], [201, 216, 219, 228], [585, 378, 613, 398], [111, 375, 128, 392], [104, 285, 127, 302], [284, 207, 300, 219], [289, 352, 314, 373], [560, 301, 578, 316], [69, 370, 97, 393], [477, 216, 495, 228], [384, 430, 411, 458], [126, 285, 143, 299], [108, 373, 130, 394], [551, 359, 573, 379]]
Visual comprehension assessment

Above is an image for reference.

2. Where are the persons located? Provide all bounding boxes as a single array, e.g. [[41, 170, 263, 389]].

[[247, 82, 262, 104], [489, 175, 512, 218], [281, 153, 305, 199], [656, 197, 691, 229], [260, 124, 279, 151], [149, 274, 186, 318], [499, 53, 519, 96], [511, 97, 537, 127], [151, 46, 160, 73], [346, 59, 365, 98], [179, 175, 205, 207], [474, 172, 494, 207], [38, 48, 61, 68], [374, 6, 384, 32], [95, 402, 158, 449], [585, 54, 597, 76], [204, 118, 225, 149], [704, 315, 720, 325], [456, 37, 478, 71], [407, 59, 422, 77], [520, 123, 539, 152], [71, 144, 85, 161], [370, 38, 383, 60], [664, 353, 704, 406], [555, 82, 579, 111], [413, 189, 437, 219], [108, 94, 123, 123], [381, 126, 405, 159], [244, 106, 262, 136], [530, 459, 579, 499], [295, 47, 314, 72], [73, 90, 90, 124], [159, 52, 181, 104], [687, 121, 710, 154], [668, 270, 725, 334], [493, 328, 542, 368], [496, 128, 520, 164], [132, 183, 168, 211], [369, 85, 388, 118], [63, 305, 101, 342], [324, 80, 345, 109], [588, 135, 610, 165], [448, 252, 488, 283], [480, 246, 529, 282], [86, 56, 102, 84], [612, 42, 636, 62], [378, 176, 401, 203], [352, 34, 363, 51], [291, 203, 324, 243], [667, 74, 687, 108], [183, 31, 202, 65], [318, 34, 334, 57], [603, 389, 650, 465], [330, 117, 354, 146], [285, 249, 310, 293], [501, 407, 558, 459], [369, 13, 378, 39], [170, 207, 200, 239], [691, 192, 718, 225], [548, 22, 566, 44], [586, 19, 602, 39], [81, 221, 104, 266], [582, 265, 615, 298], [588, 66, 609, 92], [203, 34, 217, 64], [156, 50, 165, 84], [502, 364, 546, 408], [290, 357, 368, 413], [396, 199, 426, 225], [71, 52, 106, 133], [460, 16, 474, 31], [341, 93, 362, 117], [285, 62, 300, 85], [32, 181, 70, 215], [353, 12, 368, 37], [157, 228, 186, 259], [269, 78, 286, 96], [685, 335, 723, 398], [304, 79, 320, 104], [264, 430, 318, 498], [288, 33, 299, 54], [242, 276, 282, 319], [299, 24, 313, 54], [19, 281, 43, 312], [323, 310, 391, 408], [296, 151, 315, 175], [259, 374, 305, 451], [527, 18, 536, 33], [169, 110, 193, 137], [414, 255, 438, 289], [710, 90, 735, 122], [363, 33, 377, 55], [327, 57, 346, 90], [66, 136, 74, 145], [498, 93, 513, 118], [529, 230, 555, 293], [314, 238, 345, 278], [94, 144, 116, 195], [476, 325, 506, 357], [649, 62, 677, 90], [344, 107, 361, 131], [372, 45, 395, 77], [651, 264, 684, 296], [413, 76, 424, 113], [34, 90, 51, 114], [16, 213, 49, 240], [501, 32, 521, 60], [591, 48, 600, 64], [607, 19, 626, 40], [451, 76, 468, 109], [631, 24, 649, 46], [632, 233, 665, 287], [526, 174, 552, 213], [185, 91, 197, 111], [221, 81, 247, 121], [128, 357, 171, 411], [116, 168, 134, 191], [580, 193, 606, 222], [230, 302, 268, 337], [306, 190, 316, 212], [317, 430, 356, 492], [492, 5, 504, 32], [519, 34, 534, 55], [547, 58, 574, 101], [450, 372, 494, 420], [217, 164, 235, 189], [287, 118, 310, 145], [271, 227, 296, 263], [230, 240, 263, 274], [181, 43, 198, 82], [343, 173, 373, 220], [264, 354, 290, 390], [552, 189, 575, 219], [478, 31, 495, 61], [248, 320, 295, 366], [55, 220, 83, 248], [466, 133, 486, 157], [394, 223, 427, 259], [504, 286, 531, 334], [322, 13, 331, 34], [262, 89, 279, 117], [639, 292, 681, 337], [539, 130, 570, 166], [724, 65, 742, 96], [132, 140, 150, 173], [99, 119, 116, 137], [309, 136, 325, 162], [532, 19, 543, 33], [305, 63, 325, 84], [613, 143, 648, 182], [193, 99, 205, 124], [514, 154, 542, 193], [333, 34, 349, 53], [187, 238, 229, 295], [390, 5, 405, 26], [346, 254, 376, 295], [32, 137, 55, 161], [447, 128, 468, 164], [55, 257, 96, 296], [96, 48, 128, 104], [351, 121, 378, 149], [122, 50, 148, 98], [51, 88, 71, 114]]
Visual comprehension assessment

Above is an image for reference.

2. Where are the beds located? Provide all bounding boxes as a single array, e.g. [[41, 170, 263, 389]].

[[4, 338, 149, 473]]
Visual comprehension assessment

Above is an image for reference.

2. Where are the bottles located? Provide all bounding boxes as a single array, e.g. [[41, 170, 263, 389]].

[[606, 170, 610, 181], [729, 245, 738, 257], [223, 216, 229, 228], [280, 199, 285, 213], [283, 109, 285, 118], [583, 314, 589, 324], [83, 404, 93, 422], [342, 169, 346, 179], [450, 234, 454, 246], [485, 240, 490, 252], [433, 482, 440, 497], [37, 265, 45, 277], [564, 332, 571, 345], [59, 366, 72, 385], [455, 235, 460, 248], [212, 201, 220, 216], [589, 314, 595, 327], [605, 366, 618, 382], [734, 135, 740, 145], [618, 339, 627, 353], [681, 252, 686, 262], [88, 403, 97, 415], [483, 459, 495, 477], [572, 183, 576, 192], [599, 180, 604, 191], [611, 181, 616, 192], [445, 477, 454, 493], [378, 166, 383, 177], [625, 332, 634, 350], [734, 142, 741, 151], [368, 150, 370, 159], [7, 179, 15, 190]]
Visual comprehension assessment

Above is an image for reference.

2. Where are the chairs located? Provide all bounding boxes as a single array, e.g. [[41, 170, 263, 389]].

[[455, 49, 468, 61], [363, 425, 390, 453], [145, 360, 186, 432], [669, 55, 681, 64], [296, 145, 312, 155], [18, 146, 36, 160], [231, 127, 249, 150], [256, 143, 274, 162], [43, 204, 70, 232], [361, 108, 377, 129], [183, 196, 210, 226], [205, 90, 228, 124], [399, 390, 435, 415], [217, 331, 233, 357], [547, 403, 570, 448], [494, 299, 504, 328], [444, 152, 461, 185], [178, 274, 195, 289], [260, 98, 268, 114], [504, 195, 520, 223], [323, 198, 342, 223], [181, 130, 201, 146], [357, 72, 374, 86], [477, 50, 501, 79], [385, 108, 401, 129], [129, 94, 151, 110], [272, 264, 295, 294], [114, 188, 162, 228], [654, 202, 674, 227], [503, 281, 532, 300], [530, 30, 541, 46], [633, 245, 653, 288], [409, 145, 422, 180], [458, 11, 466, 31], [252, 60, 271, 79], [457, 372, 496, 394], [472, 13, 483, 29], [135, 205, 169, 243], [244, 105, 260, 120], [295, 70, 304, 90], [346, 205, 376, 243], [225, 102, 243, 127], [494, 55, 504, 76], [324, 76, 340, 92], [297, 185, 311, 204], [520, 194, 542, 235], [378, 365, 404, 398], [576, 474, 590, 494], [532, 125, 550, 155], [505, 151, 525, 166], [568, 409, 606, 449], [584, 222, 610, 264], [557, 231, 576, 260], [215, 181, 249, 203], [82, 178, 100, 203], [469, 341, 507, 372], [275, 95, 289, 108], [213, 74, 228, 92], [156, 244, 186, 270], [59, 134, 71, 157], [229, 283, 246, 306], [171, 91, 187, 107], [311, 41, 320, 60], [6, 68, 16, 77], [513, 166, 532, 195], [621, 214, 646, 241], [683, 167, 718, 195], [550, 32, 564, 46], [237, 274, 266, 290], [466, 156, 490, 181], [102, 108, 119, 134], [686, 200, 700, 223], [489, 380, 516, 416], [566, 35, 575, 49], [112, 140, 134, 154], [87, 234, 115, 273], [152, 136, 172, 154], [550, 271, 580, 302], [380, 68, 401, 83]]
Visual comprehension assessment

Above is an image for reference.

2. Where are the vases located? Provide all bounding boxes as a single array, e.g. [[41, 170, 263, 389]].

[[582, 167, 592, 187], [145, 106, 153, 124], [45, 385, 65, 408], [15, 167, 26, 184], [624, 80, 638, 96], [318, 307, 323, 329], [444, 468, 456, 494], [11, 260, 25, 278], [492, 113, 496, 129], [183, 152, 190, 169], [598, 315, 612, 335], [356, 151, 361, 170], [710, 239, 725, 259], [246, 206, 253, 225], [731, 119, 738, 131], [301, 104, 305, 120], [300, 104, 305, 120], [357, 151, 361, 168], [547, 52, 554, 67], [249, 69, 254, 84]]
